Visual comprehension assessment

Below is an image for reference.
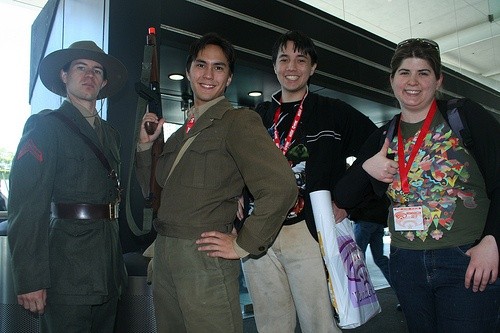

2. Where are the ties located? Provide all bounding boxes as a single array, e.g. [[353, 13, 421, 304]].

[[184, 112, 196, 137]]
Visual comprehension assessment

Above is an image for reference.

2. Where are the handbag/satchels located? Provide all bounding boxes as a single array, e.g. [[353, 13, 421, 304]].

[[317, 214, 383, 330]]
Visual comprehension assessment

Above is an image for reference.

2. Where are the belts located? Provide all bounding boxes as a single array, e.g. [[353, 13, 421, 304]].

[[50, 202, 120, 221]]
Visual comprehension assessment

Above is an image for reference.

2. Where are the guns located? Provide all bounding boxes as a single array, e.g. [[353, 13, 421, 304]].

[[133, 26, 165, 209]]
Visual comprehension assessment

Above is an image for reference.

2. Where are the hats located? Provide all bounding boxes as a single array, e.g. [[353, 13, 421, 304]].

[[39, 40, 129, 100]]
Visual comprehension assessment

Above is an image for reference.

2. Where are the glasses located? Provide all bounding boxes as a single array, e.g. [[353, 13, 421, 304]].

[[394, 38, 440, 55]]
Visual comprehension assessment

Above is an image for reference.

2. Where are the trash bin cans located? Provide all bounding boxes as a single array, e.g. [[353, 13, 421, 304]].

[[0, 220, 40, 333]]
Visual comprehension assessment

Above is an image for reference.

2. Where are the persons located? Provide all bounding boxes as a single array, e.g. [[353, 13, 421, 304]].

[[349, 193, 391, 286], [135, 32, 299, 333], [7, 41, 127, 333], [234, 30, 378, 333], [332, 37, 500, 333]]
